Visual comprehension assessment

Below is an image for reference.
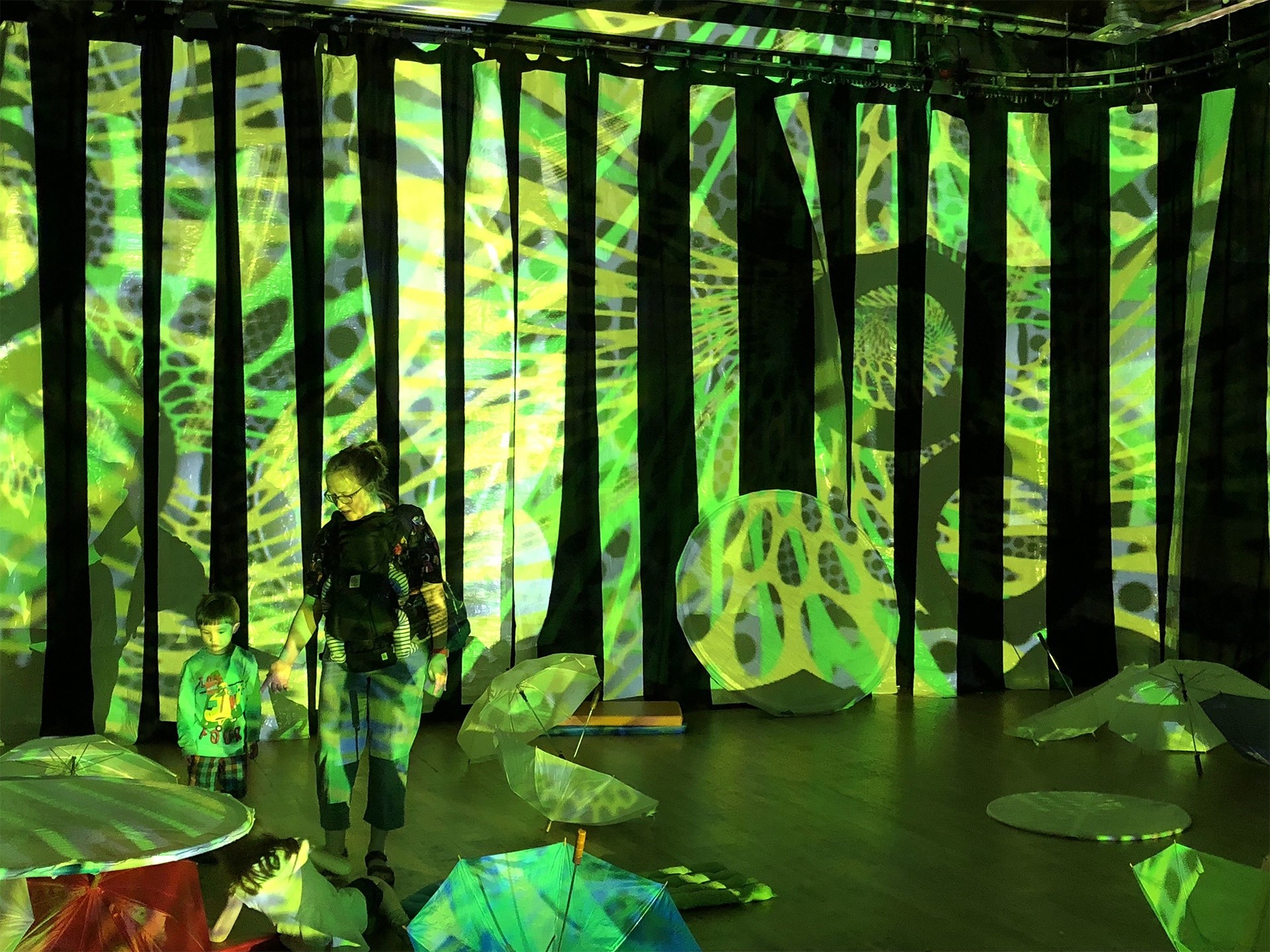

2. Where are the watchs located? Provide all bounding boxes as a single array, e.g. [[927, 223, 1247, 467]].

[[432, 648, 450, 658]]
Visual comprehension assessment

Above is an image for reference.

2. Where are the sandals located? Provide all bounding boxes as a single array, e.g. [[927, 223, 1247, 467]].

[[321, 847, 349, 877], [365, 851, 395, 888]]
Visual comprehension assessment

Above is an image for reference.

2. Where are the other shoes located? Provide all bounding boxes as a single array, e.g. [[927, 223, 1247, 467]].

[[192, 850, 219, 864]]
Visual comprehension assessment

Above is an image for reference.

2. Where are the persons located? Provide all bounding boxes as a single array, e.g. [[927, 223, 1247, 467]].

[[207, 834, 413, 952], [176, 593, 262, 865], [259, 439, 448, 889]]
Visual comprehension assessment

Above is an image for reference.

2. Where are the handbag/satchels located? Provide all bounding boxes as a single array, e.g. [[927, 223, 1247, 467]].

[[402, 582, 470, 653]]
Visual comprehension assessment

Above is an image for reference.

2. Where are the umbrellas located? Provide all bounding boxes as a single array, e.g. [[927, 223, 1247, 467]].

[[0, 734, 178, 782], [0, 859, 211, 952], [401, 829, 703, 952], [1003, 632, 1148, 748], [456, 653, 604, 765], [1129, 839, 1270, 952], [494, 687, 659, 833], [1108, 658, 1270, 777], [1196, 689, 1270, 768]]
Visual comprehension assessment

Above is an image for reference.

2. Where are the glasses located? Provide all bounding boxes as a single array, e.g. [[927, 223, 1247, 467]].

[[325, 485, 365, 505]]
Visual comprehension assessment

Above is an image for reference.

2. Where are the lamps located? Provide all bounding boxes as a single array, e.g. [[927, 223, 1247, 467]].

[[933, 46, 962, 81], [1086, 0, 1161, 46]]
